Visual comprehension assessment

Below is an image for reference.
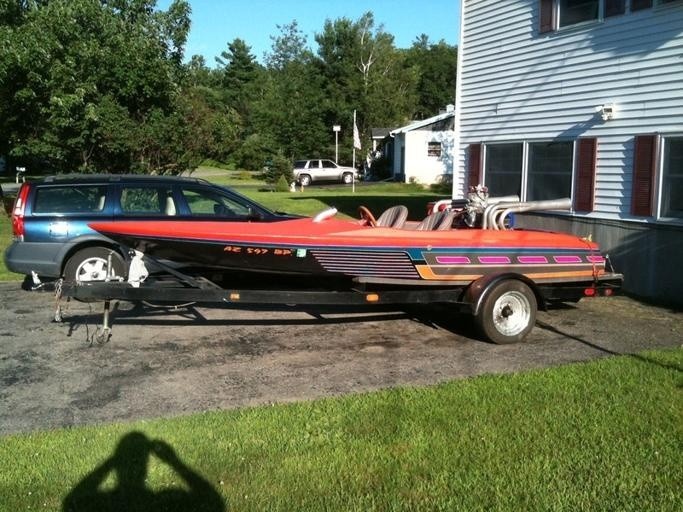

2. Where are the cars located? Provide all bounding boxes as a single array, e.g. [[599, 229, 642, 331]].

[[292, 159, 359, 187], [3, 175, 312, 302]]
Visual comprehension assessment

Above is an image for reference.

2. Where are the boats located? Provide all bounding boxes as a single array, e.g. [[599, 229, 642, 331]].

[[87, 195, 606, 286]]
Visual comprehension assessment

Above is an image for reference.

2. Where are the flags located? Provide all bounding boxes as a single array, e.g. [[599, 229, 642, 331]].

[[352, 110, 361, 151]]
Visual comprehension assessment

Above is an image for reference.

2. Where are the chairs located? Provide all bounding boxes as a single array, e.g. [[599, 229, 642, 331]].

[[164, 196, 176, 215], [93, 195, 105, 211], [377, 204, 455, 231]]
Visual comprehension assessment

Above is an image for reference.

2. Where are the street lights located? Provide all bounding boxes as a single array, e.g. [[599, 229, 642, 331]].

[[332, 125, 341, 165]]
